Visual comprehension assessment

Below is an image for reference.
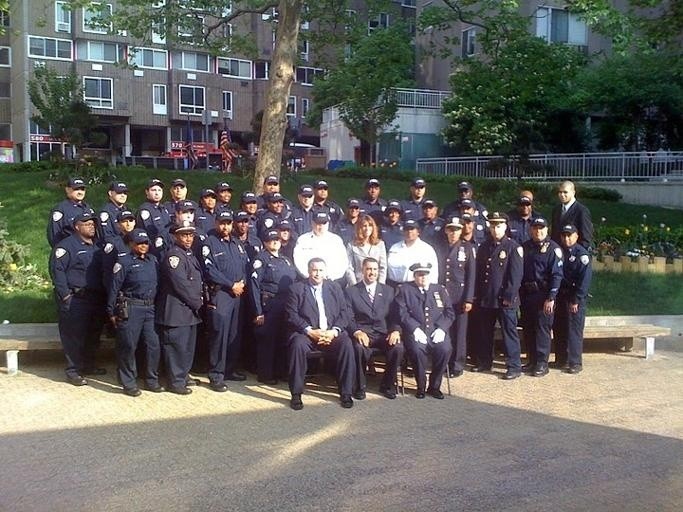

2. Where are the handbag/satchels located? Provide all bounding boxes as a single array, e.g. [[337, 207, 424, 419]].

[[116, 299, 129, 320], [201, 280, 219, 304], [523, 281, 540, 294], [259, 289, 277, 313], [70, 286, 91, 299]]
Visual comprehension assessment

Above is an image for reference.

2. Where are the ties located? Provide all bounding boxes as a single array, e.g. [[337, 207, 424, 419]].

[[560, 205, 568, 224], [423, 290, 432, 307], [313, 288, 328, 331], [368, 286, 375, 303]]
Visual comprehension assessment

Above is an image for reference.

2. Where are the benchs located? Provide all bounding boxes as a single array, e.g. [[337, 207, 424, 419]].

[[0, 321, 671, 375]]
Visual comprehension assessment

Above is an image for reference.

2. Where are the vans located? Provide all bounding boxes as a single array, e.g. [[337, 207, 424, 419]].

[[286, 142, 318, 172]]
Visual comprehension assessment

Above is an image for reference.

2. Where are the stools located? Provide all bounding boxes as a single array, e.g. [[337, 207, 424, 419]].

[[288, 350, 336, 383], [401, 346, 451, 396], [362, 346, 399, 395]]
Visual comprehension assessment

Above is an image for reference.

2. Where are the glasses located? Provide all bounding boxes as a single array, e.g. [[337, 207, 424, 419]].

[[301, 194, 312, 198], [266, 182, 277, 186]]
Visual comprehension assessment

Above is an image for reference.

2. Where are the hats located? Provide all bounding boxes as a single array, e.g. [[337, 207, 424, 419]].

[[240, 190, 257, 204], [297, 184, 314, 196], [263, 174, 280, 184], [314, 180, 328, 189], [263, 191, 288, 203], [310, 211, 331, 225], [402, 218, 419, 230], [456, 199, 476, 210], [485, 209, 510, 223], [420, 198, 439, 207], [365, 178, 381, 187], [460, 212, 474, 222], [518, 196, 531, 205], [233, 210, 252, 221], [73, 212, 98, 223], [262, 228, 281, 241], [410, 176, 426, 189], [443, 216, 465, 230], [116, 210, 136, 221], [216, 182, 232, 193], [384, 199, 403, 216], [174, 199, 196, 213], [146, 177, 164, 189], [214, 207, 234, 222], [110, 181, 128, 193], [127, 227, 150, 245], [529, 215, 549, 227], [559, 223, 578, 234], [170, 178, 186, 187], [66, 176, 86, 189], [346, 199, 359, 208], [199, 187, 217, 199], [168, 220, 198, 235], [275, 219, 291, 230], [408, 261, 432, 273], [456, 181, 472, 192]]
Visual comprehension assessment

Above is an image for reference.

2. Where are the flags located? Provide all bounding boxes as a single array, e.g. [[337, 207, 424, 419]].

[[220, 126, 236, 173]]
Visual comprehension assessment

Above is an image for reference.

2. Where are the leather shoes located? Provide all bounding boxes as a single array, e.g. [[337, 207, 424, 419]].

[[63, 373, 87, 386], [377, 386, 398, 400], [519, 362, 536, 372], [207, 383, 228, 392], [528, 365, 549, 376], [164, 384, 193, 395], [426, 388, 444, 400], [122, 388, 141, 397], [288, 393, 304, 410], [340, 393, 353, 409], [82, 366, 106, 375], [501, 370, 521, 380], [186, 378, 200, 386], [353, 388, 366, 400], [142, 382, 164, 393], [561, 365, 583, 374], [255, 374, 277, 385], [469, 365, 493, 373], [414, 386, 426, 399], [443, 370, 464, 379], [224, 371, 246, 381], [547, 361, 563, 369]]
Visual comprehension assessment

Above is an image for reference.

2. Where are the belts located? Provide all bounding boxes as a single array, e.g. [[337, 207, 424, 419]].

[[127, 299, 155, 305]]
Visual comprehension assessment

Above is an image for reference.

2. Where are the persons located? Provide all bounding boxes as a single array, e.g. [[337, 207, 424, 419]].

[[46, 175, 595, 410]]
